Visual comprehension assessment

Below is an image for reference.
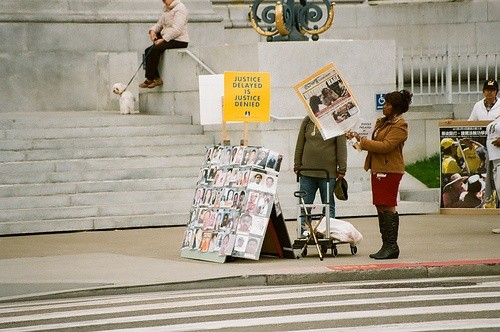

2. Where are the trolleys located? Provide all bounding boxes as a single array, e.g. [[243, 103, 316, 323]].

[[292, 168, 363, 260]]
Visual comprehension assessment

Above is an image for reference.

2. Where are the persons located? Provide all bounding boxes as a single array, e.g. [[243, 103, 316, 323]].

[[442, 172, 467, 208], [444, 79, 500, 123], [484, 114, 500, 234], [310, 95, 326, 116], [137, 0, 190, 89], [458, 136, 488, 173], [321, 88, 339, 106], [183, 146, 284, 254], [440, 137, 462, 174], [292, 115, 347, 239], [348, 88, 414, 260], [460, 174, 485, 208]]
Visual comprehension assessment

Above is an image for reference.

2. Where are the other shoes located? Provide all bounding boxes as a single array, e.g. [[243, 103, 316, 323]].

[[138, 78, 155, 88], [148, 78, 162, 88], [492, 228, 500, 234]]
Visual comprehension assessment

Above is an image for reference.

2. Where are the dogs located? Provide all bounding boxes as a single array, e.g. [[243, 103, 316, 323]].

[[113, 83, 135, 115]]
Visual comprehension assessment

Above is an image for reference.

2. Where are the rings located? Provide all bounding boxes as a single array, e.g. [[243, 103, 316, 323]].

[[353, 133, 354, 134]]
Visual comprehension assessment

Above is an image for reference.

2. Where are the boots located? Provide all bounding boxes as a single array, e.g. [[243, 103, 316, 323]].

[[368, 210, 387, 258], [375, 209, 400, 260]]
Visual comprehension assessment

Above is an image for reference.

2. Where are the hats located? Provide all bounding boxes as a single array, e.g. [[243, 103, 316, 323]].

[[385, 90, 414, 114], [483, 79, 499, 91], [468, 174, 480, 184], [443, 172, 468, 188], [334, 177, 349, 201], [440, 138, 459, 150]]
